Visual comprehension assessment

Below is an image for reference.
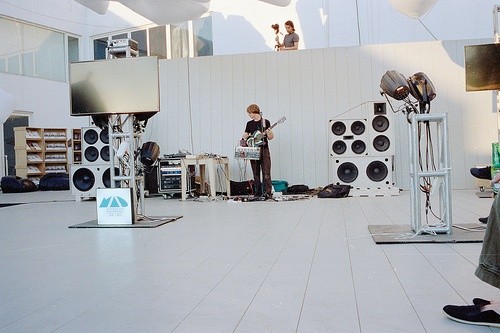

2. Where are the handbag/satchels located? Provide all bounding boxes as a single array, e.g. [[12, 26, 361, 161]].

[[317, 182, 351, 198]]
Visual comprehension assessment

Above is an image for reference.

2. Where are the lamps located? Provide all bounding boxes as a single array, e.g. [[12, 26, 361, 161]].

[[378, 67, 437, 229], [135, 141, 161, 178]]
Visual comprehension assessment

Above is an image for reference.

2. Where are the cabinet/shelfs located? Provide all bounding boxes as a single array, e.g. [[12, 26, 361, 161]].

[[27, 127, 45, 178], [43, 127, 68, 175], [72, 129, 83, 164]]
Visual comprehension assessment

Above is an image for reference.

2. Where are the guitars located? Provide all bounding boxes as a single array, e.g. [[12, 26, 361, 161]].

[[246, 116, 287, 147]]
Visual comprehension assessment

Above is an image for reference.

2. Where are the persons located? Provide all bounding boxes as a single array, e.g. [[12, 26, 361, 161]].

[[241, 105, 274, 200], [443, 165, 500, 327], [275, 21, 299, 50]]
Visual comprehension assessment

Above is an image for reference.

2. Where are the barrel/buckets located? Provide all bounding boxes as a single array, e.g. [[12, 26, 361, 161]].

[[271, 180, 287, 191]]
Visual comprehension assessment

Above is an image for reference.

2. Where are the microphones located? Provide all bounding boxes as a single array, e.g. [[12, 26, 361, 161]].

[[259, 111, 262, 116]]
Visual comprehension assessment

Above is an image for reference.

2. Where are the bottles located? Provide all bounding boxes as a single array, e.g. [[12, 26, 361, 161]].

[[490, 142, 500, 196]]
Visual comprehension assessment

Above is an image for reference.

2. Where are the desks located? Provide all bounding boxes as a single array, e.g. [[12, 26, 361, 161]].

[[182, 155, 229, 202]]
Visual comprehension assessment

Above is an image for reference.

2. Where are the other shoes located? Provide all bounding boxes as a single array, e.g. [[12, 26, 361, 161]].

[[479, 217, 488, 223], [470, 166, 492, 180], [473, 298, 491, 307], [443, 305, 500, 326]]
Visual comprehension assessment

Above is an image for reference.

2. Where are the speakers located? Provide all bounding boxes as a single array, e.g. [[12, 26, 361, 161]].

[[329, 101, 395, 197], [69, 126, 129, 197]]
